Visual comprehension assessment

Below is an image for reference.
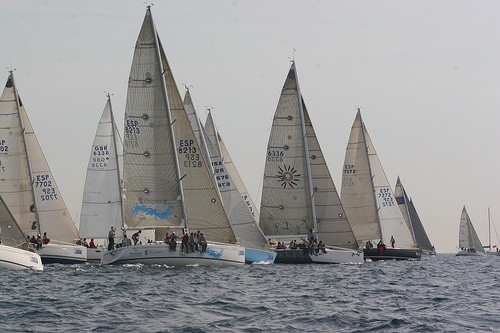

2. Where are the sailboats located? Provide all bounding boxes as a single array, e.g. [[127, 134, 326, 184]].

[[394, 176, 436, 255], [455, 205, 487, 258], [0, 68, 87, 264], [0, 193, 44, 272], [486, 208, 500, 257], [79, 93, 155, 263], [182, 85, 277, 266], [340, 108, 421, 261], [102, 5, 245, 265], [259, 60, 364, 265]]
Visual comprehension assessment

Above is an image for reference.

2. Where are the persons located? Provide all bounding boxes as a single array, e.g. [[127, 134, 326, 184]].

[[365, 236, 395, 252], [24, 232, 51, 249], [107, 227, 141, 252], [164, 231, 207, 254], [267, 228, 327, 255], [77, 238, 96, 248]]
